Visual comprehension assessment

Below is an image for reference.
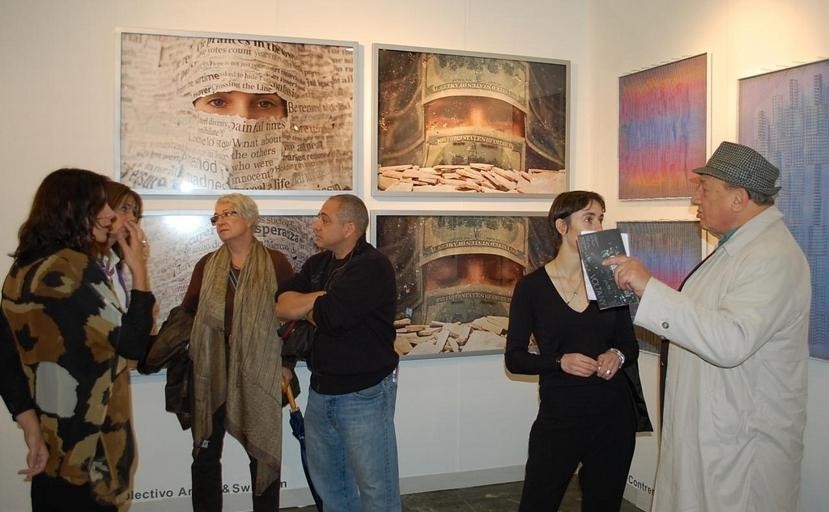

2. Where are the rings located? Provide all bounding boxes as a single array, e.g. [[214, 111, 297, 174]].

[[141, 240, 148, 245], [605, 369, 612, 374]]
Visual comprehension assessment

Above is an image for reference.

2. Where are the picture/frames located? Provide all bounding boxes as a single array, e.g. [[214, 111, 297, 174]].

[[612, 218, 708, 360], [116, 29, 367, 204], [367, 207, 557, 364], [731, 57, 828, 364], [618, 48, 715, 205], [118, 209, 322, 338], [369, 41, 574, 204]]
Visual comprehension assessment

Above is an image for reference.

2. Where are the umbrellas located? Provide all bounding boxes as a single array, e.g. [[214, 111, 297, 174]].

[[281, 375, 323, 511]]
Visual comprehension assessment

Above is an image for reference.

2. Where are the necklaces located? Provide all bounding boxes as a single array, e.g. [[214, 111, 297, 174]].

[[553, 260, 584, 303]]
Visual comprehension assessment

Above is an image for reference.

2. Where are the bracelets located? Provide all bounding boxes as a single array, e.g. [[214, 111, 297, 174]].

[[607, 346, 626, 368]]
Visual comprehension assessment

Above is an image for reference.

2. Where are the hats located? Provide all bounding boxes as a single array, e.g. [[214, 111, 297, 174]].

[[691, 140, 782, 196]]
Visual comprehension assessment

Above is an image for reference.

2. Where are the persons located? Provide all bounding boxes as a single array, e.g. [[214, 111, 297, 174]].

[[193, 90, 288, 121], [503, 188, 655, 510], [1, 167, 155, 511], [181, 195, 300, 512], [419, 254, 523, 293], [423, 95, 525, 139], [274, 194, 402, 511], [603, 140, 812, 511], [96, 180, 142, 327]]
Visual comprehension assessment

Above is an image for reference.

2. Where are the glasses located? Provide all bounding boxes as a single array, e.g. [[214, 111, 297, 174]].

[[210, 210, 238, 226]]
